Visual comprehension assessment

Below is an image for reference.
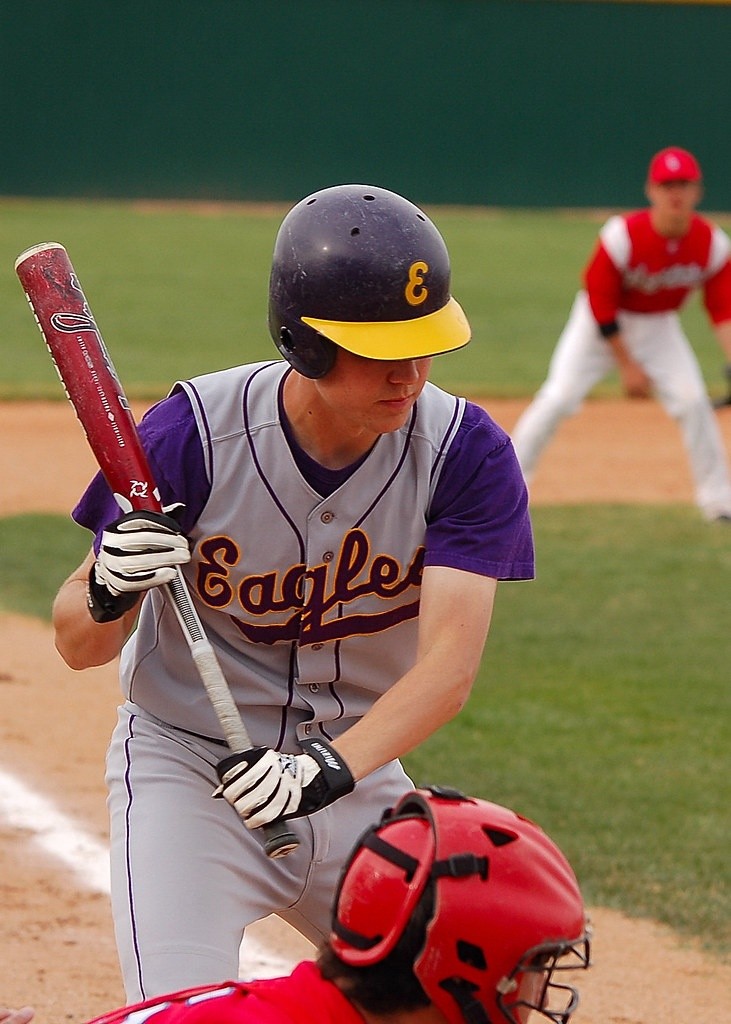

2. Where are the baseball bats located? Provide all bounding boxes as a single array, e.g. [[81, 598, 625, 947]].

[[16, 241, 303, 862]]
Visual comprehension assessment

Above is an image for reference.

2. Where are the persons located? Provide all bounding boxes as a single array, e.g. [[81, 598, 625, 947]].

[[509, 148, 731, 519], [53, 184, 534, 1007], [86, 784, 591, 1023]]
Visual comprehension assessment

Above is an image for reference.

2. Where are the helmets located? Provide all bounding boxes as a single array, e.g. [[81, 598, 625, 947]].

[[268, 184, 472, 379], [329, 789, 587, 1024]]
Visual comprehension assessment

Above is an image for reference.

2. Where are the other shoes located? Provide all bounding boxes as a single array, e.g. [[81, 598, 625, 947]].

[[703, 502, 729, 520]]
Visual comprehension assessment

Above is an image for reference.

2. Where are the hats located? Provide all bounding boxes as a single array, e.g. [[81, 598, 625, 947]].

[[649, 147, 701, 184]]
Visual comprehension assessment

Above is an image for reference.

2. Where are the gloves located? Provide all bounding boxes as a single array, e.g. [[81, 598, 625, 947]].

[[211, 736, 354, 829], [86, 510, 189, 624]]
